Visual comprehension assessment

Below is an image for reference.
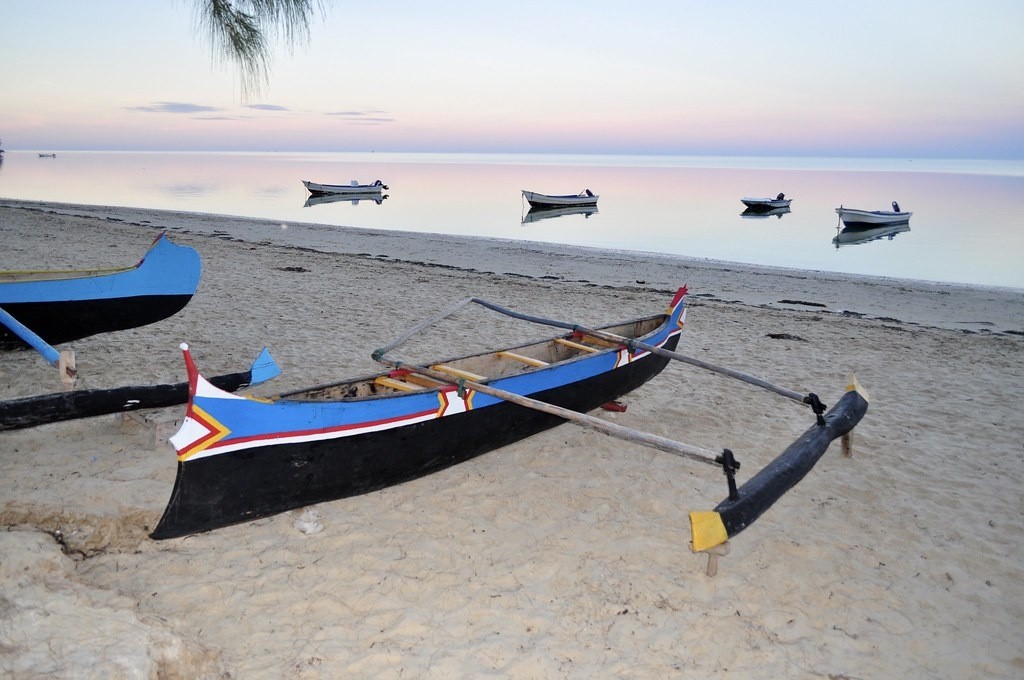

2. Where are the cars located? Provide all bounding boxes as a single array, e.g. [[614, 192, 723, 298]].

[[149, 282, 692, 541]]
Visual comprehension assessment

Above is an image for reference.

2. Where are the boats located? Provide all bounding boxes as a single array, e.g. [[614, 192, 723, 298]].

[[520, 206, 600, 224], [740, 193, 794, 207], [303, 194, 389, 207], [739, 207, 791, 218], [521, 188, 601, 204], [831, 225, 911, 247], [301, 178, 390, 193], [834, 201, 913, 225], [0, 227, 202, 353]]
